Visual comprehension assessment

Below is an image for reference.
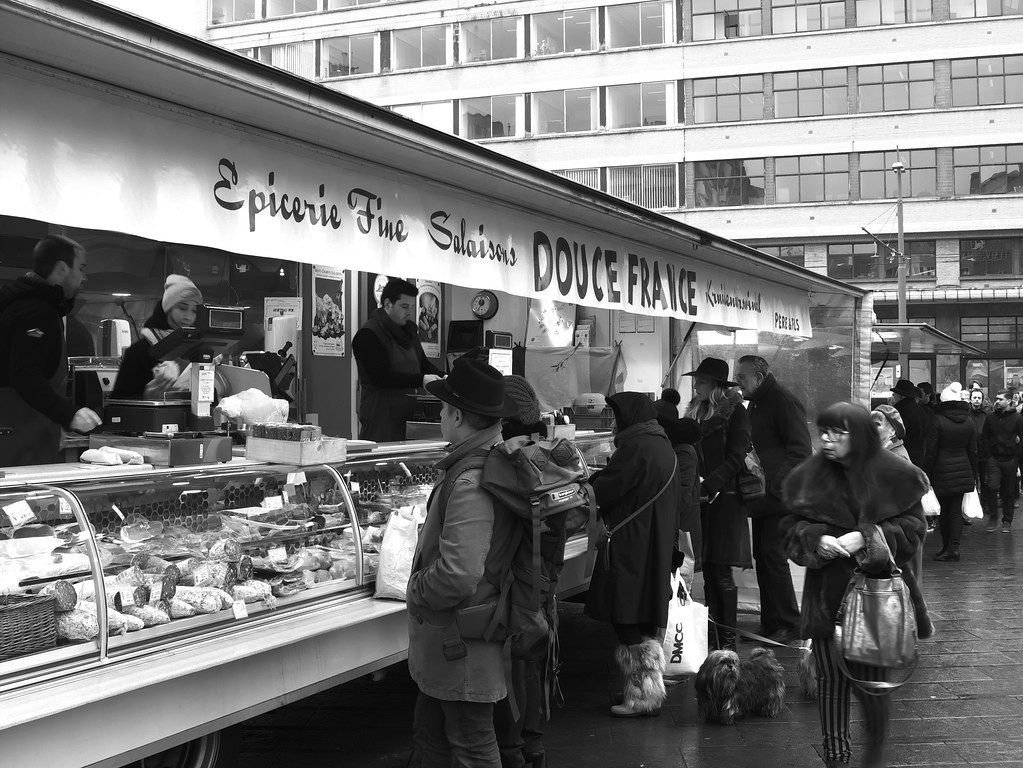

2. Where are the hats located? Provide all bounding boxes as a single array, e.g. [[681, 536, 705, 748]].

[[425, 358, 520, 418], [874, 403, 906, 440], [890, 379, 916, 400], [162, 274, 203, 314], [502, 374, 540, 426], [653, 389, 681, 419], [681, 356, 739, 387], [940, 381, 962, 402]]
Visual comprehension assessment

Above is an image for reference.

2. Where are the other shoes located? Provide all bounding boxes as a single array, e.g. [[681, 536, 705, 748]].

[[1014, 499, 1020, 507], [766, 627, 800, 646], [925, 511, 1011, 533], [740, 620, 781, 641]]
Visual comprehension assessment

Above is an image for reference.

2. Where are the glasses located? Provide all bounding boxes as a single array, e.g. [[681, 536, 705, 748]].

[[816, 425, 850, 443], [732, 371, 756, 383]]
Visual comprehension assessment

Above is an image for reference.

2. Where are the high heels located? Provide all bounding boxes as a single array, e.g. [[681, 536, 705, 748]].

[[934, 541, 959, 561]]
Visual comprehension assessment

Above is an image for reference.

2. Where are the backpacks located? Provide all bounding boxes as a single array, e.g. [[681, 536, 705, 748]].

[[439, 436, 600, 606]]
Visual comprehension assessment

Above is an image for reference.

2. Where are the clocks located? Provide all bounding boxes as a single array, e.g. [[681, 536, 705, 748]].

[[471, 289, 500, 321]]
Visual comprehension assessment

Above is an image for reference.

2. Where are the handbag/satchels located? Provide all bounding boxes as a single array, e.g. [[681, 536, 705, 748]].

[[920, 485, 941, 517], [961, 484, 983, 524], [832, 522, 918, 694], [594, 521, 611, 571], [723, 403, 766, 501]]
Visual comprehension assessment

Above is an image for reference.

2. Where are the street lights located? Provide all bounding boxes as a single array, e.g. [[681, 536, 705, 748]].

[[890, 144, 910, 381]]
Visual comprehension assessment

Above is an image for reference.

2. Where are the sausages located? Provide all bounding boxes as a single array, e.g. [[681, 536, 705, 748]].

[[80, 446, 144, 464], [36, 540, 272, 641]]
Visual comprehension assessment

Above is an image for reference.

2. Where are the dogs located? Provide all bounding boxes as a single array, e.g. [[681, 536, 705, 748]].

[[691, 645, 789, 728], [794, 637, 822, 701]]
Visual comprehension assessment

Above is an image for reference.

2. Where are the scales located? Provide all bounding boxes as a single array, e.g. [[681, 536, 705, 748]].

[[405, 329, 514, 422], [101, 324, 245, 439]]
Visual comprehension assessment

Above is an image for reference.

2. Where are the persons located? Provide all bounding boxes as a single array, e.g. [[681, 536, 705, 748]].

[[654, 388, 701, 684], [407, 359, 508, 768], [352, 277, 450, 444], [681, 358, 752, 653], [494, 374, 558, 768], [587, 391, 679, 716], [0, 234, 102, 467], [733, 356, 812, 647], [109, 274, 215, 399], [775, 401, 936, 768], [871, 405, 923, 589], [887, 380, 1023, 561]]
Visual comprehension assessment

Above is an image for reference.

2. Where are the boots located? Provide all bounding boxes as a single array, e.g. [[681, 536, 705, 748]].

[[609, 639, 666, 717], [704, 585, 741, 652]]
